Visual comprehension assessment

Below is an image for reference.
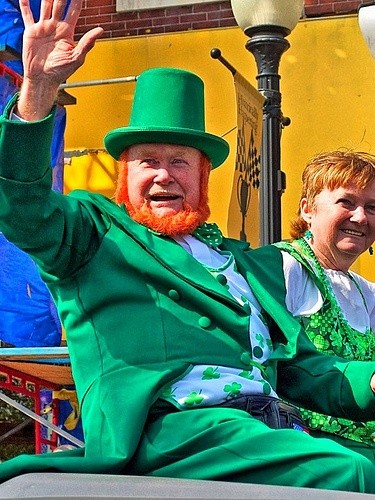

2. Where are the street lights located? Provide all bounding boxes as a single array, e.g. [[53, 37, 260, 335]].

[[230, 0, 306, 253]]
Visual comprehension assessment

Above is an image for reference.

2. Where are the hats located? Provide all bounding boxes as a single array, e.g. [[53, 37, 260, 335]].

[[103, 68, 230, 170]]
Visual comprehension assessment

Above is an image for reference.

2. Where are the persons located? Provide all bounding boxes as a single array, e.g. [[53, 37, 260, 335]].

[[0, 0, 375, 494], [270, 146, 375, 466]]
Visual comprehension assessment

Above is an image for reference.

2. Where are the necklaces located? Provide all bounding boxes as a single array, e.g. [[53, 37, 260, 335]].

[[298, 237, 372, 361]]
